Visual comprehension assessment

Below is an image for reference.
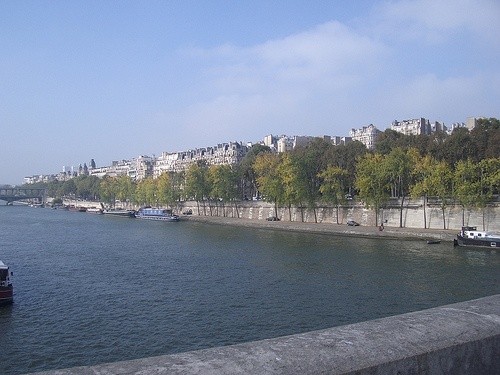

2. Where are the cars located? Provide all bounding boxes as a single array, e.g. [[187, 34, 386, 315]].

[[347, 221, 359, 226], [266, 217, 280, 221]]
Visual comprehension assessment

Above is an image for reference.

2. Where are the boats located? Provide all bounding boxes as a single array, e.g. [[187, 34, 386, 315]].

[[103, 207, 136, 217], [453, 226, 500, 249], [135, 206, 179, 222]]
